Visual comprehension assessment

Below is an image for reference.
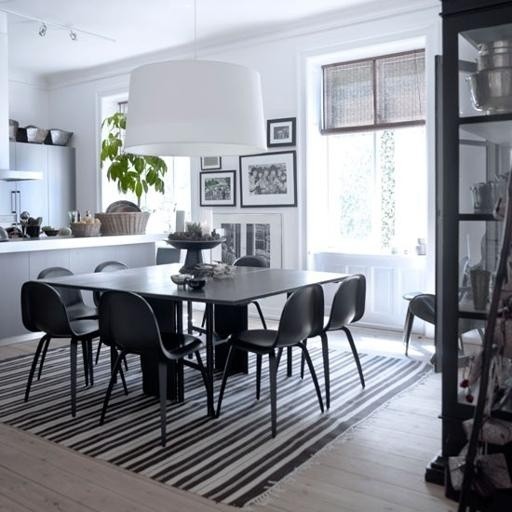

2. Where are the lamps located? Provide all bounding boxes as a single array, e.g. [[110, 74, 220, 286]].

[[123, 60, 267, 157], [39, 27, 46, 35], [70, 32, 75, 40]]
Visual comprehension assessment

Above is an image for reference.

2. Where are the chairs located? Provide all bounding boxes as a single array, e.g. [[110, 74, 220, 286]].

[[403, 257, 486, 357], [37, 268, 128, 387], [100, 291, 208, 447], [300, 274, 367, 411], [20, 280, 127, 417], [199, 257, 267, 338], [95, 262, 129, 371], [216, 284, 325, 438]]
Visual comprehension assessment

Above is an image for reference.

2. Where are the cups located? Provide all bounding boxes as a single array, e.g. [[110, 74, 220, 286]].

[[30, 226, 40, 238]]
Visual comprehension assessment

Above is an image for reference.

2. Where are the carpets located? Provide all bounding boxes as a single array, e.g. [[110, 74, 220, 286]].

[[1, 330, 435, 508]]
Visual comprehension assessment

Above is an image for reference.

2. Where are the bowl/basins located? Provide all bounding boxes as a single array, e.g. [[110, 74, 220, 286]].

[[45, 230, 60, 236], [186, 279, 206, 289], [171, 274, 191, 285]]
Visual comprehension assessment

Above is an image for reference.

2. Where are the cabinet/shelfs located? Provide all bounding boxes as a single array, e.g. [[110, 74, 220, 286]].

[[425, 0, 512, 486], [48, 145, 75, 236], [0, 141, 49, 236]]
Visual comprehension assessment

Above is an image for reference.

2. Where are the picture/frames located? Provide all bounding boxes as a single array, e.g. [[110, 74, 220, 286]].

[[200, 170, 236, 207], [212, 213, 282, 270], [239, 151, 296, 208], [200, 157, 221, 170], [267, 117, 297, 148]]
